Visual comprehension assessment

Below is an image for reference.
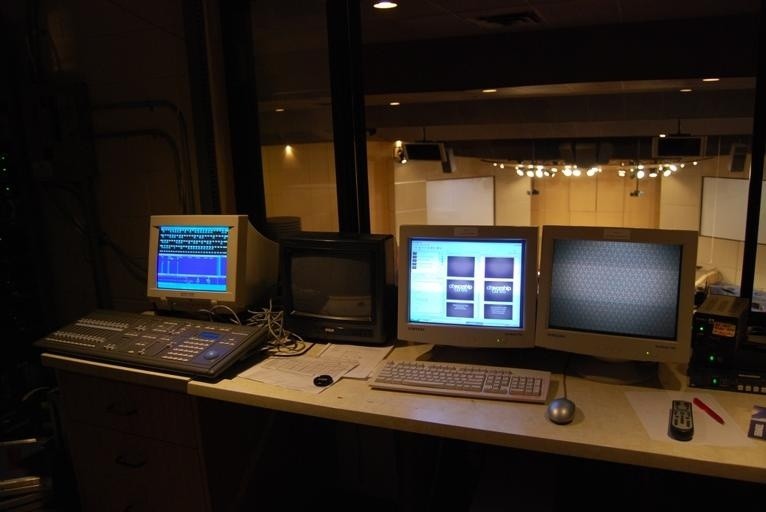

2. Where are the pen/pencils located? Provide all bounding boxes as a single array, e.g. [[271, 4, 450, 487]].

[[693, 397, 724, 425], [315, 342, 332, 358]]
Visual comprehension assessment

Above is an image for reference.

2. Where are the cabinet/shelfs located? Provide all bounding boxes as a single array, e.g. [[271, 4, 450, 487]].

[[41, 349, 275, 512]]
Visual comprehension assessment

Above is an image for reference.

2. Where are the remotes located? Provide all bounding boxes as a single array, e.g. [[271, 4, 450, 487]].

[[670, 399, 695, 439]]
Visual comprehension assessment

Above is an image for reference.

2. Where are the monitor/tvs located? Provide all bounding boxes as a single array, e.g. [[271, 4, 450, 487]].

[[534, 225, 699, 385], [397, 224, 539, 369], [280, 230, 395, 345], [146, 214, 280, 316]]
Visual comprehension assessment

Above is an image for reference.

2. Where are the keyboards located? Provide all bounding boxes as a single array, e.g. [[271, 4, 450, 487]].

[[368, 359, 551, 403]]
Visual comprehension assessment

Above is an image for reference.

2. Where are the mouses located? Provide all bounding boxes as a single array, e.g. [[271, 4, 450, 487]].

[[548, 398, 575, 423]]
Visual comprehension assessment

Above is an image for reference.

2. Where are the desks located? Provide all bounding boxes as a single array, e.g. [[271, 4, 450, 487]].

[[188, 338, 766, 511]]
